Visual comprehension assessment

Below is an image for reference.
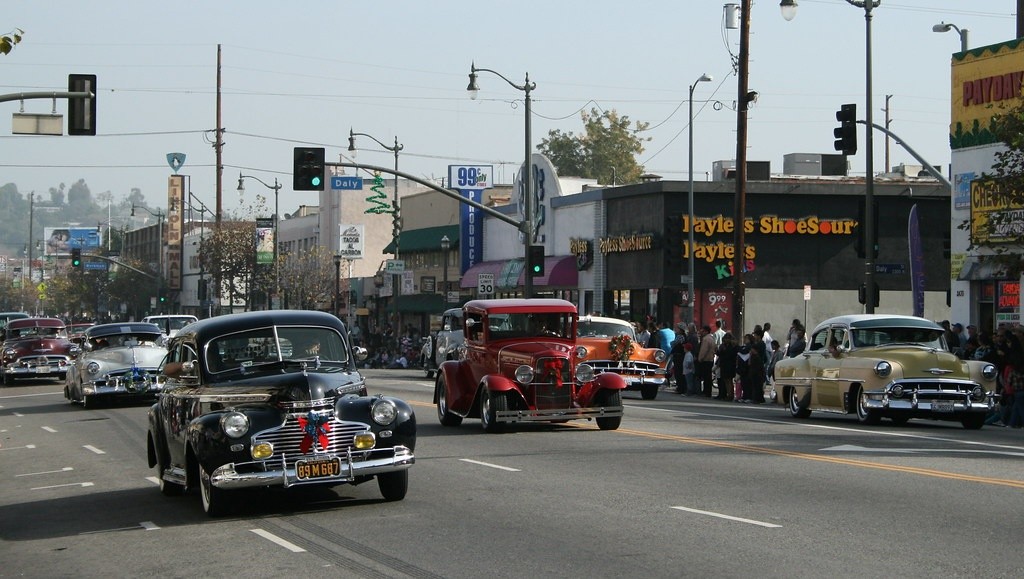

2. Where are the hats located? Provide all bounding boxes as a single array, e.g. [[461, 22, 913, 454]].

[[682, 343, 693, 350], [677, 322, 687, 330], [967, 324, 977, 332], [942, 320, 949, 325], [752, 329, 764, 337], [952, 323, 962, 331]]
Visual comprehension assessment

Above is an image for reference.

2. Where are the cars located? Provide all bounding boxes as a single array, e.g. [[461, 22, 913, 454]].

[[0, 318, 82, 386], [772, 312, 1001, 429], [420, 308, 512, 379], [432, 298, 625, 435], [0, 312, 30, 340], [64, 321, 169, 410], [66, 323, 97, 344], [147, 308, 418, 516], [560, 316, 667, 399]]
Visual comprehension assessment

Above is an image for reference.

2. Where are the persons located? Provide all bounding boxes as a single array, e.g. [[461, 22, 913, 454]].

[[627, 314, 808, 404], [47, 230, 70, 252], [350, 321, 423, 369], [825, 329, 864, 358], [937, 320, 1024, 428], [288, 334, 329, 360], [536, 320, 561, 338]]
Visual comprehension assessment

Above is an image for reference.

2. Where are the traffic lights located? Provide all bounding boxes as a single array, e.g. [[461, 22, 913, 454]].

[[529, 246, 545, 277], [293, 147, 324, 190], [833, 103, 857, 155], [72, 248, 81, 267]]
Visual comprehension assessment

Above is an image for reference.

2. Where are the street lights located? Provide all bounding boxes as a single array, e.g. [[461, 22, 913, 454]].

[[130, 202, 161, 313], [171, 199, 206, 317], [21, 238, 60, 318], [466, 64, 534, 299], [348, 131, 401, 355], [687, 71, 717, 321], [332, 255, 341, 319], [236, 175, 278, 295], [97, 217, 125, 261], [441, 234, 451, 302], [932, 21, 968, 52], [727, 0, 800, 339]]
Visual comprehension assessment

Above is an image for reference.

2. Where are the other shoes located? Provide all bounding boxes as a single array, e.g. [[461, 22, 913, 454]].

[[739, 398, 753, 403], [993, 421, 1005, 427], [682, 393, 690, 397], [724, 397, 732, 402], [1006, 424, 1020, 428], [714, 394, 723, 400]]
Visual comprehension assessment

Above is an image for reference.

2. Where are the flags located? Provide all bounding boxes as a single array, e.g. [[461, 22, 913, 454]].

[[909, 204, 924, 318]]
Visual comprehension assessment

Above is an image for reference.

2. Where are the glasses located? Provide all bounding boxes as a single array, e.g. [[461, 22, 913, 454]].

[[996, 334, 1005, 338]]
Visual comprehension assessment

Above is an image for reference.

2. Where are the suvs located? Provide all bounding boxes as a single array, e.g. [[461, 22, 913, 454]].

[[142, 315, 199, 343]]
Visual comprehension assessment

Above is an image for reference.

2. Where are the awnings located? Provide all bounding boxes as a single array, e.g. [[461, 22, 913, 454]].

[[956, 254, 1022, 282], [459, 254, 579, 288], [382, 224, 459, 255], [385, 293, 452, 313]]
